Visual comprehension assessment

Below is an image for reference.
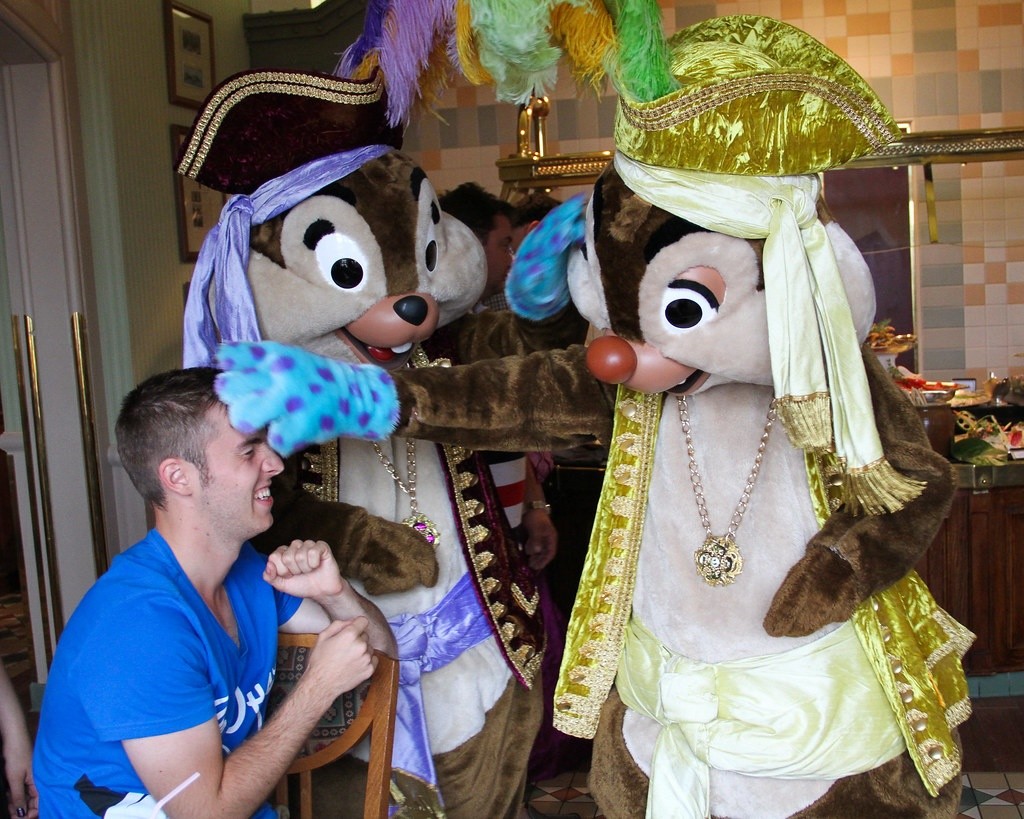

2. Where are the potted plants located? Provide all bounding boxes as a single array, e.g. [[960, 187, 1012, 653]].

[[866, 319, 920, 372]]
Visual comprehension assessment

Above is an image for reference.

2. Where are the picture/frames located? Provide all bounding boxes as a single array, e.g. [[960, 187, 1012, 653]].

[[162, 0, 216, 112], [169, 124, 238, 266]]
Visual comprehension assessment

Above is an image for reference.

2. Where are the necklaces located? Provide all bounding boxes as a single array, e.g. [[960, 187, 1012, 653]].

[[674, 389, 779, 589], [366, 437, 443, 557]]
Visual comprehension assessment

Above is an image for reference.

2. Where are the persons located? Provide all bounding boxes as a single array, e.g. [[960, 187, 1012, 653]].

[[437, 180, 561, 572], [484, 191, 564, 311], [29, 363, 400, 819], [0, 662, 40, 819]]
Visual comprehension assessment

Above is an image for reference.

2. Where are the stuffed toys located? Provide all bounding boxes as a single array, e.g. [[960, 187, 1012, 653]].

[[170, 66, 563, 819], [210, 0, 981, 819]]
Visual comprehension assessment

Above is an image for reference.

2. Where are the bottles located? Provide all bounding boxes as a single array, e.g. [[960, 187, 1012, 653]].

[[913, 404, 955, 460]]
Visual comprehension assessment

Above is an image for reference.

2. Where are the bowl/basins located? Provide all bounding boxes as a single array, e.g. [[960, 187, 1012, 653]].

[[992, 382, 1010, 399], [923, 390, 956, 403], [870, 336, 916, 354]]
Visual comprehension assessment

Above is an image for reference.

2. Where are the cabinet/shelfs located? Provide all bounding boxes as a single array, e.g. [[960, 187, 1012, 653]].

[[910, 466, 1024, 677]]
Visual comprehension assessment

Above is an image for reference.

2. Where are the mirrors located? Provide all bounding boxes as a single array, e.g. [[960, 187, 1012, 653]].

[[821, 122, 923, 373]]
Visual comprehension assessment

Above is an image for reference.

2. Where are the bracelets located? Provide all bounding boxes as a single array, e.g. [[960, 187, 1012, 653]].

[[522, 499, 554, 515]]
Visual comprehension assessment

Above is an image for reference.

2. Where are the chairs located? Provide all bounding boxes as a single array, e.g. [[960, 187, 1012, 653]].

[[261, 631, 400, 819]]
